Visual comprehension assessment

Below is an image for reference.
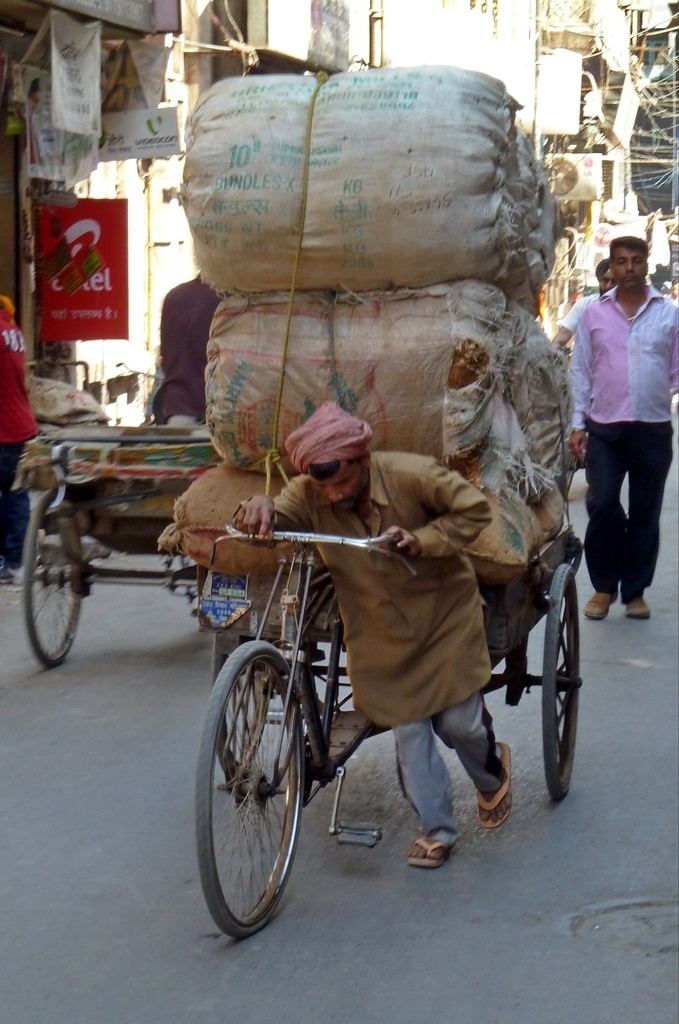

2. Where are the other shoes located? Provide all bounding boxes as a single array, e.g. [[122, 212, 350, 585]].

[[582, 591, 618, 618], [623, 598, 651, 619], [0, 556, 26, 585]]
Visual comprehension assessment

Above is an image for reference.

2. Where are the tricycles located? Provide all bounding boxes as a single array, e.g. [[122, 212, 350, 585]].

[[196, 519, 583, 937], [22, 412, 223, 671]]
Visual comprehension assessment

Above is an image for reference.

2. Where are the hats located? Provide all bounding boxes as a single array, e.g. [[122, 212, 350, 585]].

[[284, 400, 374, 475]]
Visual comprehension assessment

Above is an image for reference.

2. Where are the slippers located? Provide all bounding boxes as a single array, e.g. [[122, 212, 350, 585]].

[[406, 837, 448, 867], [475, 741, 513, 828]]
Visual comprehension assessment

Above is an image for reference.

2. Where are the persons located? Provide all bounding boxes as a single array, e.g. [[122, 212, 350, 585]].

[[0, 296, 40, 584], [232, 402, 513, 868], [153, 272, 222, 431], [546, 237, 679, 619]]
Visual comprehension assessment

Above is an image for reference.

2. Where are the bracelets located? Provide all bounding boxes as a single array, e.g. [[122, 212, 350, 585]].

[[571, 429, 578, 434]]
